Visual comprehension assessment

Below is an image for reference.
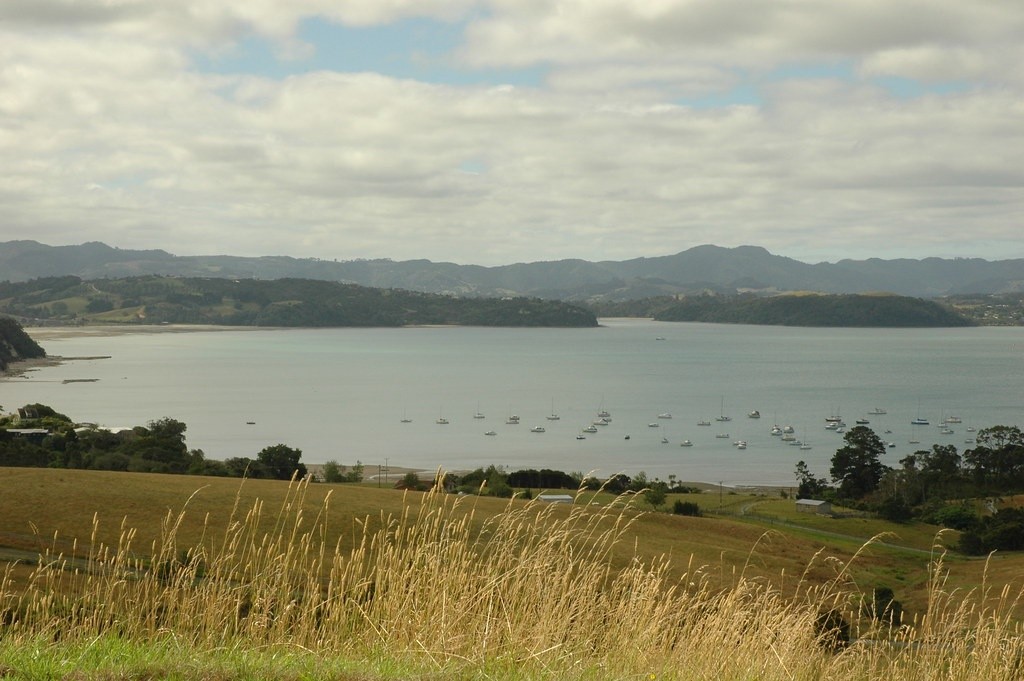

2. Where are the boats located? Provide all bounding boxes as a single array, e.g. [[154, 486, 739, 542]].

[[399, 403, 984, 452]]
